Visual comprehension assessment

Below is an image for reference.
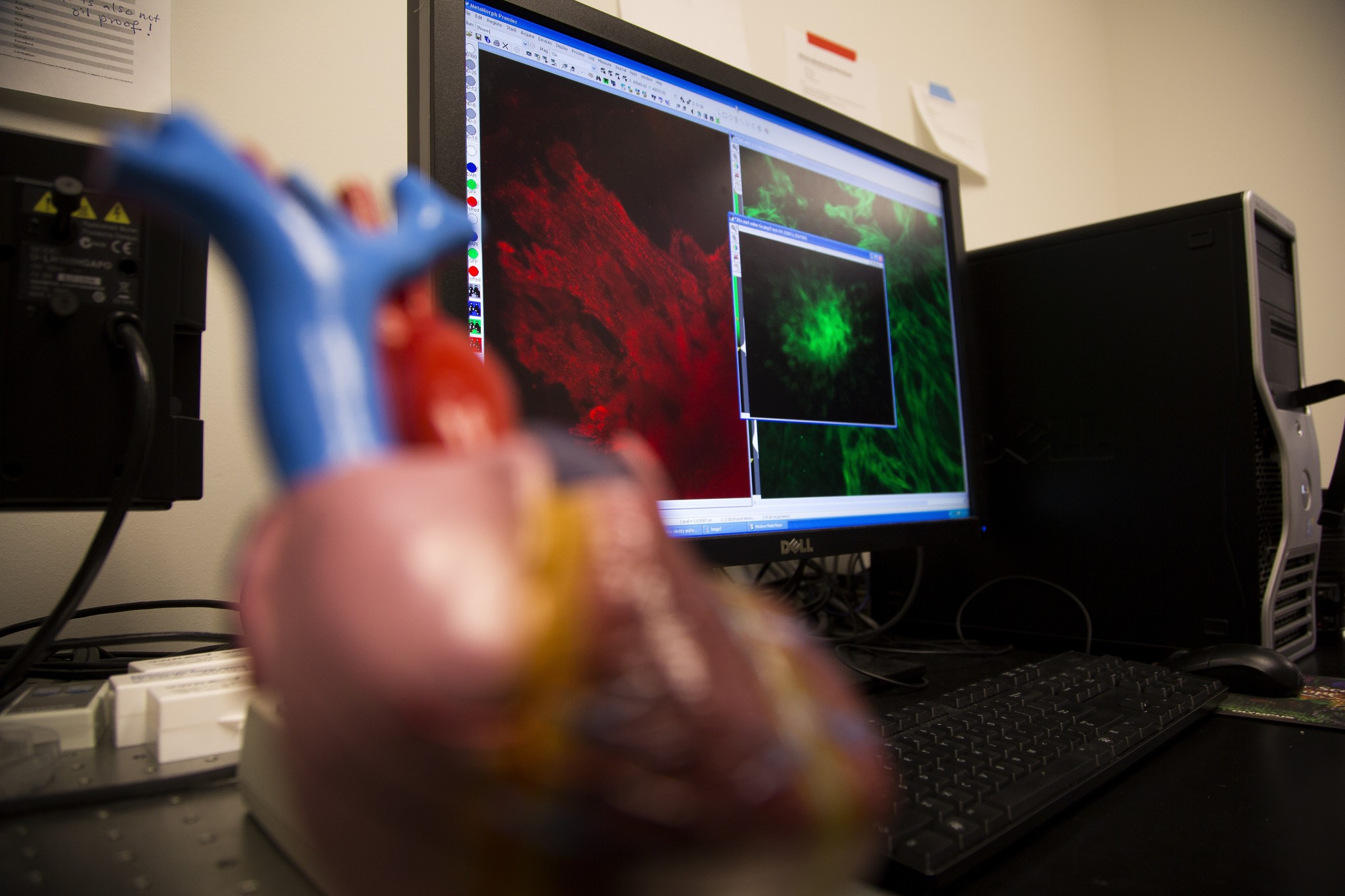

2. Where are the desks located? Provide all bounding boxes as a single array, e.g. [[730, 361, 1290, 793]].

[[0, 643, 1345, 896]]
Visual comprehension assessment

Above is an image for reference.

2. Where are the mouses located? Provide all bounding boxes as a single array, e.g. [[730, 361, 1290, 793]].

[[1166, 643, 1301, 698]]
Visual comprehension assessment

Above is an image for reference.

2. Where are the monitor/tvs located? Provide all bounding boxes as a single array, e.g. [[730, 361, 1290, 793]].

[[407, 0, 974, 538], [0, 89, 210, 516]]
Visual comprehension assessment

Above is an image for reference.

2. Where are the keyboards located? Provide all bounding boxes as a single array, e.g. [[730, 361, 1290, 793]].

[[861, 646, 1225, 890]]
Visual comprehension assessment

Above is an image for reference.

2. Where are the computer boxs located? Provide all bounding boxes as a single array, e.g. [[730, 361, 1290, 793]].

[[869, 187, 1316, 665]]
